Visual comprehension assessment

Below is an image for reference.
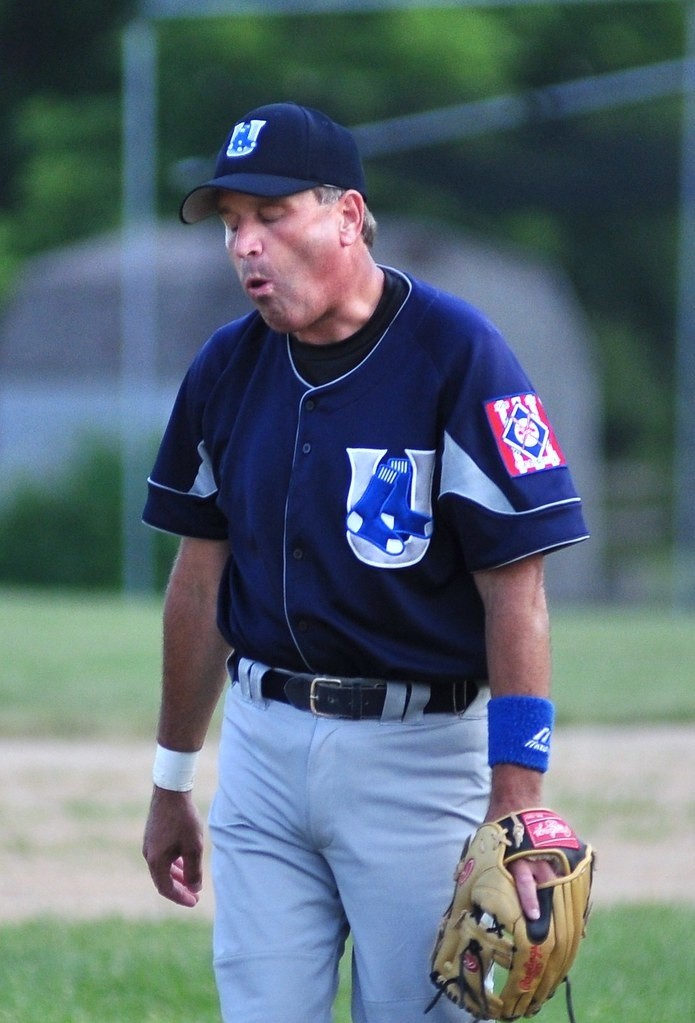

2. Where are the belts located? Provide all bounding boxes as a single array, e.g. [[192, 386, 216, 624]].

[[233, 657, 481, 720]]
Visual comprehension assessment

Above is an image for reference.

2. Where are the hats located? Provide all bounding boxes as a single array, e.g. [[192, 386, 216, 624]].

[[179, 101, 368, 227]]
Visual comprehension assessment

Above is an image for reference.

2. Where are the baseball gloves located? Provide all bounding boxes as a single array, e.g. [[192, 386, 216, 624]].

[[423, 806, 606, 1023]]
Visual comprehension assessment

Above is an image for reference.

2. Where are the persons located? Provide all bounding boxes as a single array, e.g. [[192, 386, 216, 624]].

[[142, 103, 589, 1023]]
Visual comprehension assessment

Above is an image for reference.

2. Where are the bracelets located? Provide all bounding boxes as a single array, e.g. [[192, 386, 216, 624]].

[[153, 744, 202, 792], [487, 698, 553, 772]]
